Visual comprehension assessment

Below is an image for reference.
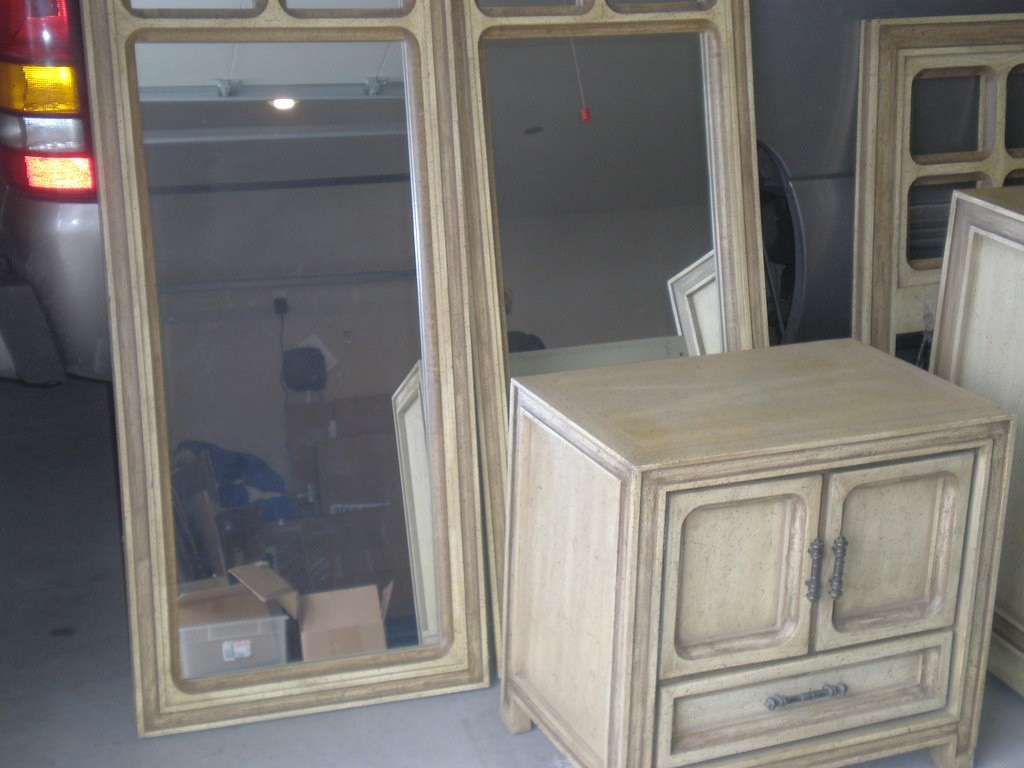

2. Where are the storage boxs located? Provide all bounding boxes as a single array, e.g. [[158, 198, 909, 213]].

[[171, 395, 416, 681]]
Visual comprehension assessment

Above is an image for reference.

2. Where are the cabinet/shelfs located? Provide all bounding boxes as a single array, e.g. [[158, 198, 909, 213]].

[[928, 185, 1024, 704], [496, 338, 1023, 767]]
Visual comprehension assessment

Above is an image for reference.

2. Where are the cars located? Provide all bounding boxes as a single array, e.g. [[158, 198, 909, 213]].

[[1, 0, 1024, 593]]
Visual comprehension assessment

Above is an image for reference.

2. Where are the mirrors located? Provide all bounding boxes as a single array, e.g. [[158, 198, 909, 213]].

[[451, 0, 770, 675], [78, 1, 488, 740]]
